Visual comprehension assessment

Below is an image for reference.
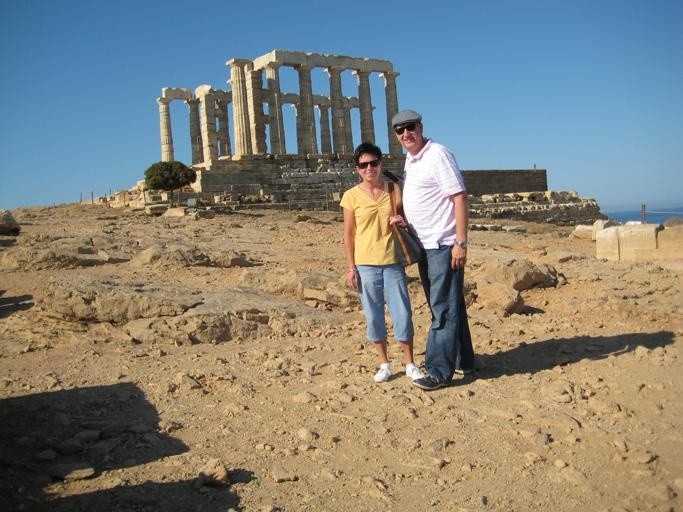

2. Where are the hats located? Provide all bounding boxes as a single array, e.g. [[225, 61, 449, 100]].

[[391, 109, 422, 127]]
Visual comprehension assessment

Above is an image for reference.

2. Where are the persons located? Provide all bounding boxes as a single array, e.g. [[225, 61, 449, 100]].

[[338, 142, 426, 385], [388, 109, 475, 391]]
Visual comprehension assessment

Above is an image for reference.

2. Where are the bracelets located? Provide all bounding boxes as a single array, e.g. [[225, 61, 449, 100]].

[[452, 237, 468, 248], [346, 269, 357, 273]]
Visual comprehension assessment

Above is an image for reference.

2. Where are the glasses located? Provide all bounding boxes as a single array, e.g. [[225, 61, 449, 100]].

[[357, 160, 379, 170], [394, 123, 416, 136]]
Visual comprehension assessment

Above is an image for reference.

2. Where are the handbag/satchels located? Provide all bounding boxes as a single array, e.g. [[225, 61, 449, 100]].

[[387, 182, 423, 267]]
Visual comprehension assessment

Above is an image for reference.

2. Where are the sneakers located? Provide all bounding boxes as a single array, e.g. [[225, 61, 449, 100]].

[[412, 373, 450, 391], [373, 363, 391, 383], [406, 363, 426, 380], [455, 365, 472, 374]]
